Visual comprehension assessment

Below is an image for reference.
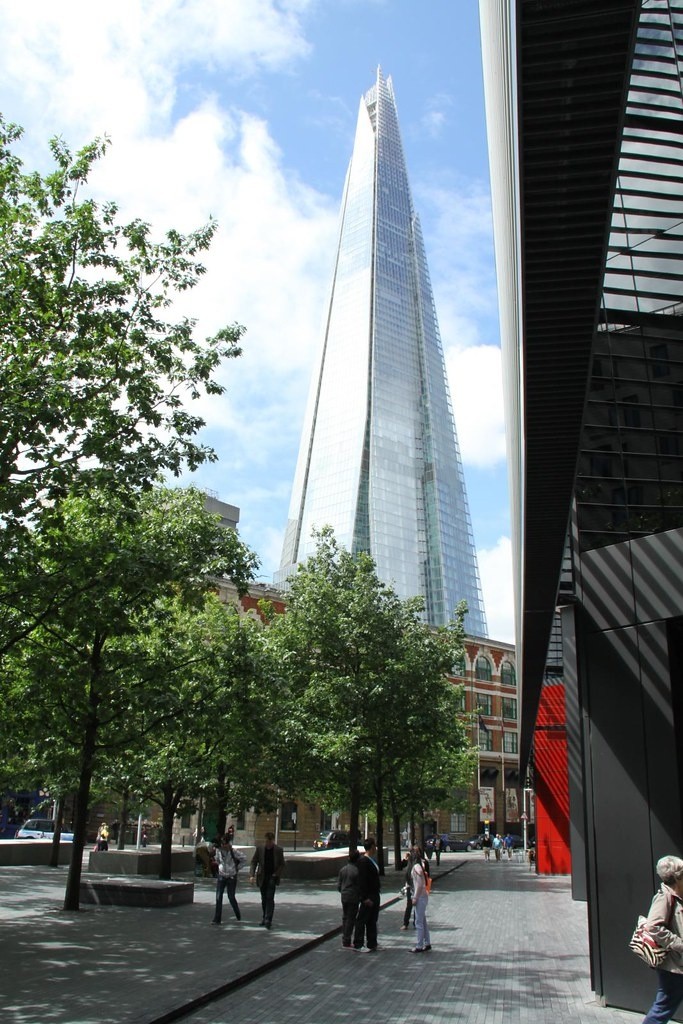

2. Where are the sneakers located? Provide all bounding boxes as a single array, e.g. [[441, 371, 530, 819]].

[[374, 944, 385, 951], [343, 944, 354, 949], [353, 946, 370, 952]]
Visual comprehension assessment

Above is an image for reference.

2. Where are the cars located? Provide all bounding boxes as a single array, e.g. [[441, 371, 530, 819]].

[[16, 818, 74, 842], [425, 833, 473, 852], [528, 835, 536, 846], [466, 834, 495, 849], [502, 835, 531, 849], [313, 830, 351, 850]]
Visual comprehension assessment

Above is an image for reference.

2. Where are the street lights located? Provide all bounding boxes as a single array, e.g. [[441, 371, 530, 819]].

[[523, 788, 534, 851]]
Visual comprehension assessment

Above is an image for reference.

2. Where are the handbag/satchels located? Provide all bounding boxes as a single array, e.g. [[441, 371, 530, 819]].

[[628, 895, 676, 970], [424, 872, 431, 894]]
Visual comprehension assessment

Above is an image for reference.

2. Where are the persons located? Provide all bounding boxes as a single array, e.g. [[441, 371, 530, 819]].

[[249, 832, 285, 929], [433, 834, 444, 866], [641, 856, 683, 1024], [481, 834, 492, 862], [352, 838, 385, 952], [211, 830, 221, 877], [211, 835, 242, 926], [401, 828, 408, 848], [493, 834, 503, 862], [400, 844, 432, 952], [504, 833, 514, 860], [337, 850, 360, 949], [96, 822, 109, 851]]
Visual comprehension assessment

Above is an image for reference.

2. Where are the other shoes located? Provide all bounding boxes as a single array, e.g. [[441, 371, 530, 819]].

[[400, 925, 407, 930], [211, 920, 221, 925], [237, 914, 241, 920], [265, 923, 271, 929], [259, 921, 265, 925], [409, 946, 423, 953], [423, 945, 431, 951]]
